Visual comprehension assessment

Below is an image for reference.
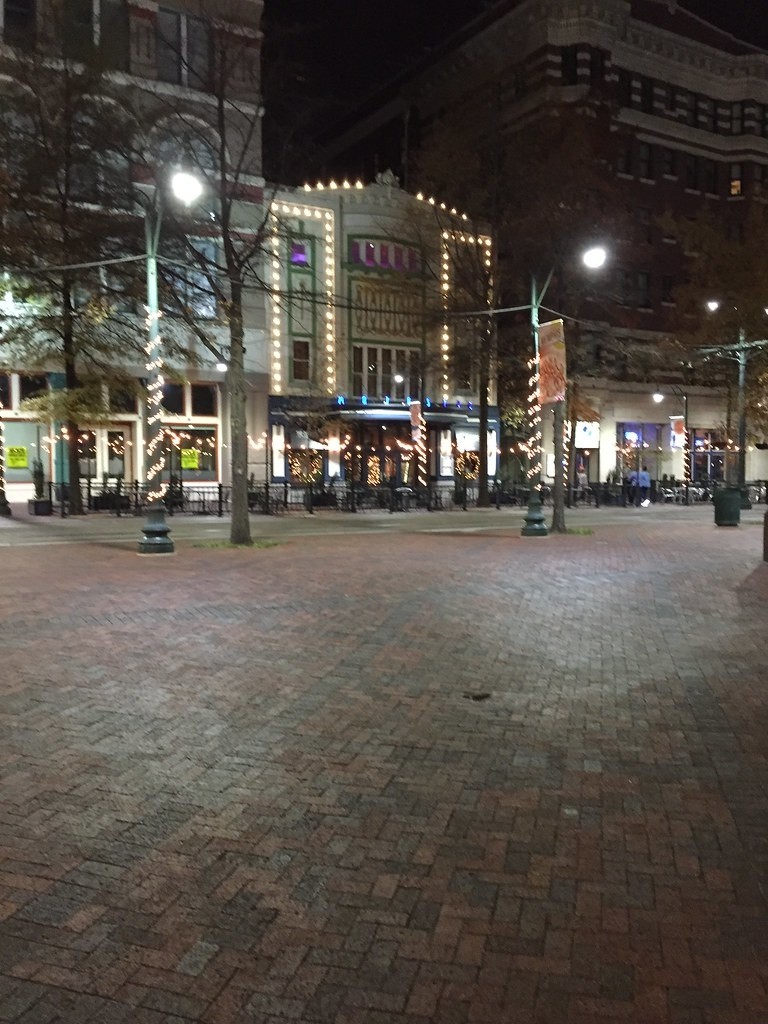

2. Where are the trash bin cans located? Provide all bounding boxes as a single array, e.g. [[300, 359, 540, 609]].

[[715, 487, 741, 525]]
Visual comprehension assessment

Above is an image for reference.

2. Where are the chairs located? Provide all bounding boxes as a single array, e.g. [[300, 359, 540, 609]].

[[49, 480, 765, 516]]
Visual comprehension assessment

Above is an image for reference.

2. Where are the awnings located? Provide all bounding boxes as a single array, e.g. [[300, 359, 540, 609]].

[[327, 409, 471, 429]]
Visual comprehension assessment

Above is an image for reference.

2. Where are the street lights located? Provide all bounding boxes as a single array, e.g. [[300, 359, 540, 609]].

[[520, 246, 609, 538], [135, 170, 205, 557]]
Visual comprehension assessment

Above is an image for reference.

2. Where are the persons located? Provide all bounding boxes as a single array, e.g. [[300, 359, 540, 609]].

[[538, 481, 552, 503], [662, 473, 680, 498], [394, 483, 413, 508], [162, 476, 182, 506], [576, 465, 591, 502], [626, 470, 638, 505], [641, 467, 650, 507]]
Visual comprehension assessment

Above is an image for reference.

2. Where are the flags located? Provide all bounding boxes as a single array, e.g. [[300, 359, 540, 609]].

[[670, 415, 688, 450], [536, 317, 568, 404]]
